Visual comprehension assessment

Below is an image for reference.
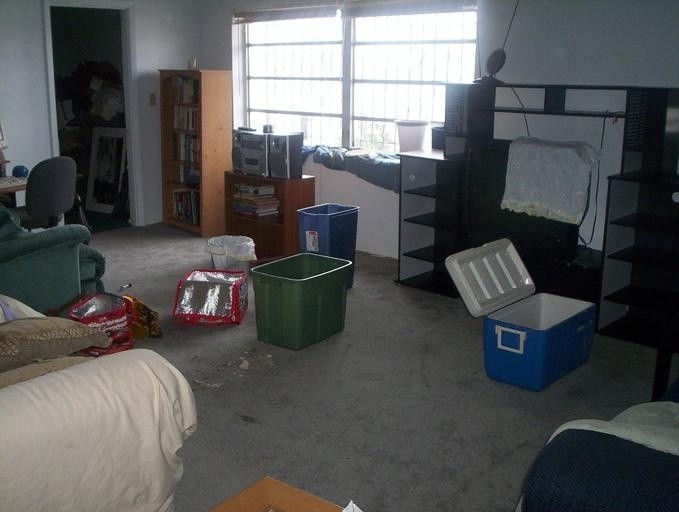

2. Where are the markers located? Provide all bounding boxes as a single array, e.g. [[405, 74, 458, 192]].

[[120, 283, 132, 292]]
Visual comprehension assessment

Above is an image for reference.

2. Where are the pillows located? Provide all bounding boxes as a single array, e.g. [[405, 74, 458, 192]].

[[0, 316, 109, 373]]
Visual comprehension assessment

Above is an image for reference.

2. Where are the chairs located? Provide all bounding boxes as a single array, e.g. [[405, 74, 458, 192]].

[[0, 209, 105, 316], [0, 295, 198, 511], [514, 401, 678, 512], [12, 156, 77, 232]]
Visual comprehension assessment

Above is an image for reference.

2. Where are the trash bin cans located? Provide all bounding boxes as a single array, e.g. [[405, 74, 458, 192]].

[[207, 234, 254, 278]]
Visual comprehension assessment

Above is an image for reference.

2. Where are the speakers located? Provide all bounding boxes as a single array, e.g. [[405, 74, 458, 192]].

[[269, 133, 303, 179], [232, 129, 257, 171]]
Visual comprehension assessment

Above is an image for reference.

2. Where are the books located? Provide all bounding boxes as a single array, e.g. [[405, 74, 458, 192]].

[[232, 182, 280, 216], [172, 76, 200, 225]]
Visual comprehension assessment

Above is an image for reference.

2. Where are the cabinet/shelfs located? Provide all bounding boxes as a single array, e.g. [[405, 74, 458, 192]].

[[394, 84, 679, 351], [158, 69, 233, 238], [224, 170, 315, 256]]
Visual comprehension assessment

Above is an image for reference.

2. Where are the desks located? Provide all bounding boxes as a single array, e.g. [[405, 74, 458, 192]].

[[0, 176, 28, 209]]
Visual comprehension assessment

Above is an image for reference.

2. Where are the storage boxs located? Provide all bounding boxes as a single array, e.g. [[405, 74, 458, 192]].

[[250, 253, 353, 351], [444, 238, 596, 392]]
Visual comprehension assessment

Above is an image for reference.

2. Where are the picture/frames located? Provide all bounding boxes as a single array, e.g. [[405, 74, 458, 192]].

[[86, 127, 126, 214]]
